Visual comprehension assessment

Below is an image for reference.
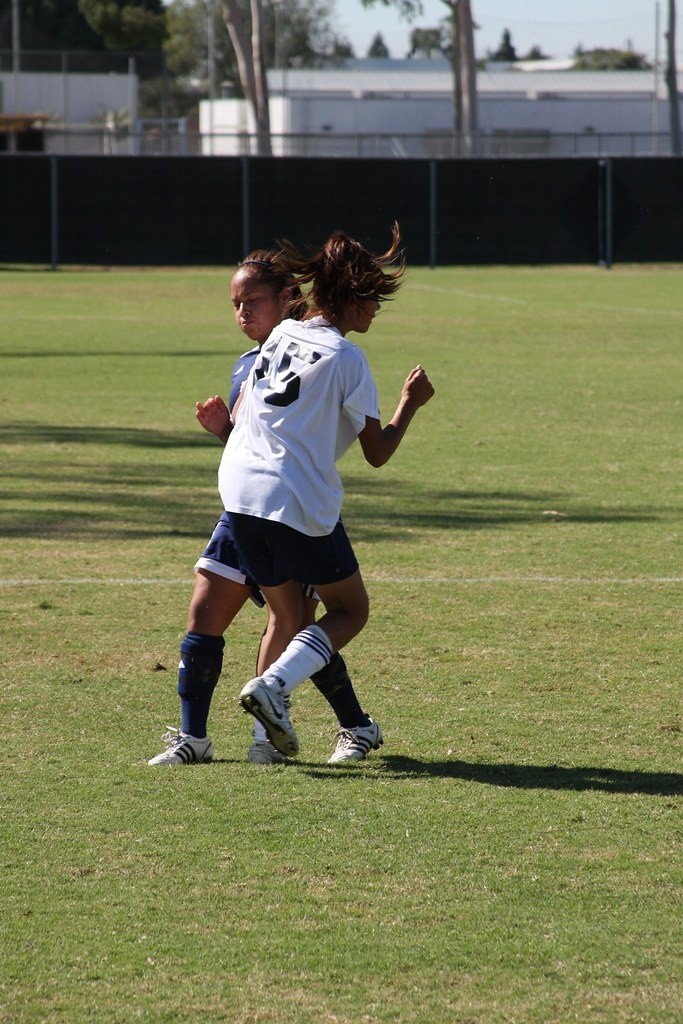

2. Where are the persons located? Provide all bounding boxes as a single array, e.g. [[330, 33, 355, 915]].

[[148, 250, 383, 768], [218, 226, 435, 762]]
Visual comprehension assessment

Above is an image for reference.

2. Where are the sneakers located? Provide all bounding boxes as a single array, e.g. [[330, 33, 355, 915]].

[[238, 676, 300, 758], [146, 726, 214, 768], [327, 715, 384, 767], [245, 741, 288, 765]]
